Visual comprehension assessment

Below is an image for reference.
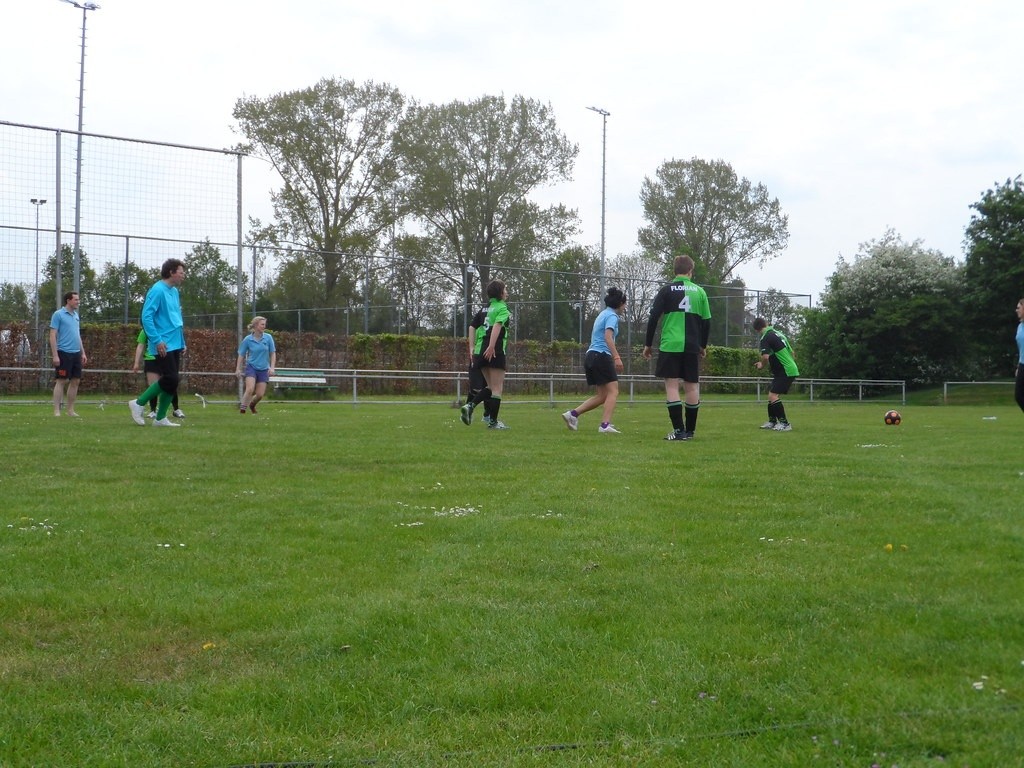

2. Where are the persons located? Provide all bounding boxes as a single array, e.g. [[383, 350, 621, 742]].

[[642, 254, 712, 441], [562, 286, 628, 434], [460, 279, 512, 430], [128, 257, 190, 428], [235, 315, 277, 415], [1014, 298, 1024, 413], [752, 317, 800, 432], [49, 291, 88, 418]]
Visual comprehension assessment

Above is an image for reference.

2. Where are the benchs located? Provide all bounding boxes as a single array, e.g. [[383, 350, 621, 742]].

[[269, 371, 339, 397]]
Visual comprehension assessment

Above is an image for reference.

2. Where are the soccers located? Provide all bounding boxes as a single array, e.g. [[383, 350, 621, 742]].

[[884, 410, 901, 425]]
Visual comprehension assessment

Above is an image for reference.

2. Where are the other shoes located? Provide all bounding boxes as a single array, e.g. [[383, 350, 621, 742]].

[[147, 411, 157, 418], [664, 429, 695, 440], [487, 421, 510, 429], [482, 417, 490, 422], [760, 422, 793, 431], [598, 424, 621, 434], [173, 408, 185, 418], [461, 404, 473, 426], [151, 417, 181, 428], [561, 410, 578, 431], [128, 399, 146, 425], [249, 405, 257, 416]]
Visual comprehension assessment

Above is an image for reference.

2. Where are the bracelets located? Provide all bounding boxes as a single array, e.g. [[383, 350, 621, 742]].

[[613, 356, 622, 359]]
